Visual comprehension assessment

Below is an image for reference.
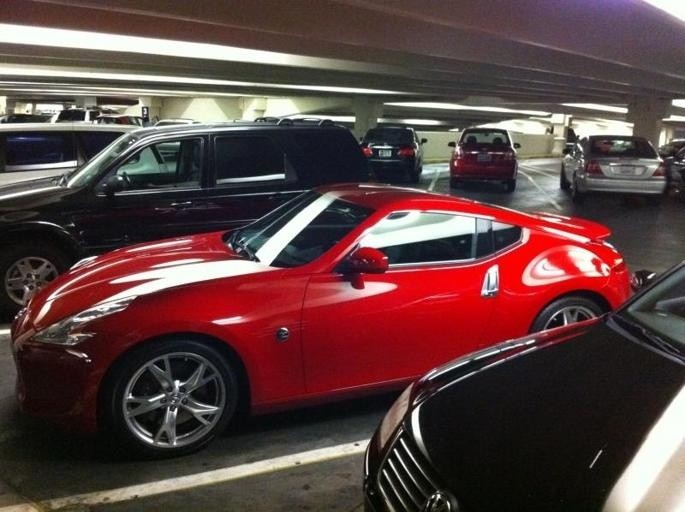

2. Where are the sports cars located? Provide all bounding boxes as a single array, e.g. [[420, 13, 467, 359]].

[[8, 181, 659, 461]]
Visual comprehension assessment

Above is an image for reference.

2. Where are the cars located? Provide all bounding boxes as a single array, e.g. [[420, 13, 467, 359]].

[[656, 139, 685, 201], [447, 128, 522, 193], [357, 125, 429, 186], [362, 253, 685, 511], [560, 135, 668, 204], [545, 126, 581, 151]]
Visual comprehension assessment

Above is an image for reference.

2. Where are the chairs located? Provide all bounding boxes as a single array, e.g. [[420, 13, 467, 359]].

[[467, 136, 476, 144], [493, 138, 503, 145]]
